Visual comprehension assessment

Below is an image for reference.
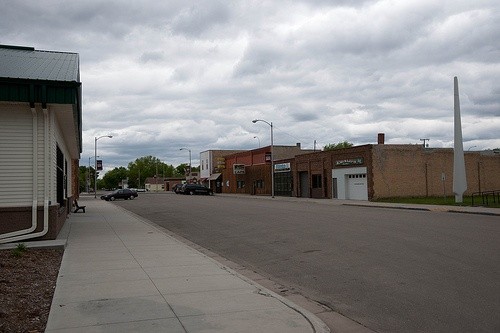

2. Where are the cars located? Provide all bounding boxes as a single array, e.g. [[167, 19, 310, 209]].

[[101, 189, 139, 202], [172, 183, 213, 196]]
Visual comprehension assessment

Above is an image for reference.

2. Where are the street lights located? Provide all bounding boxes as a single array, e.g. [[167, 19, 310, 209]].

[[251, 119, 274, 199], [179, 148, 192, 184], [254, 136, 260, 148], [89, 156, 100, 196], [95, 135, 113, 198]]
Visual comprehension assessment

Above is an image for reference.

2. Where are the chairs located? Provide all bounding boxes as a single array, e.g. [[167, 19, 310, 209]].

[[74, 198, 86, 213]]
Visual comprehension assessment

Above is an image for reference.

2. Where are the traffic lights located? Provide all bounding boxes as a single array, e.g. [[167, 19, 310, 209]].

[[96, 172, 99, 179]]
